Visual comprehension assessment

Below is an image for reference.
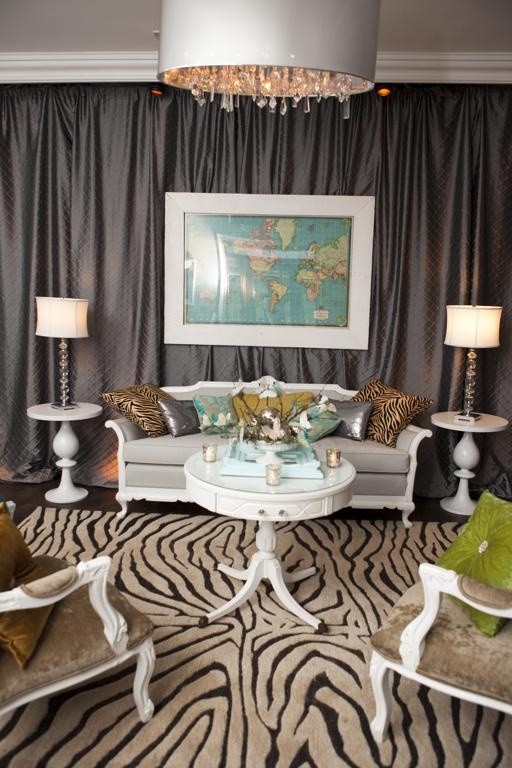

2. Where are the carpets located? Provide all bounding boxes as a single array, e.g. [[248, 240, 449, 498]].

[[0, 505, 512, 768]]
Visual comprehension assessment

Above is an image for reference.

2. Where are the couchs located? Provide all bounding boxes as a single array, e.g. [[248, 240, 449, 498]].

[[104, 381, 431, 528]]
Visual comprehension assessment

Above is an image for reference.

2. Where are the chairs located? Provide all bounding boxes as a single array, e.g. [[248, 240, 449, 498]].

[[0, 500, 155, 724], [368, 562, 512, 744]]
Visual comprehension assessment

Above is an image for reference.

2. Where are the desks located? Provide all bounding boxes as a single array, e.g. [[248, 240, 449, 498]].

[[184, 445, 356, 632]]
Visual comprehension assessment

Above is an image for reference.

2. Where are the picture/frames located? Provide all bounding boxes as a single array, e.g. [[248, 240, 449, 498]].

[[164, 192, 374, 352]]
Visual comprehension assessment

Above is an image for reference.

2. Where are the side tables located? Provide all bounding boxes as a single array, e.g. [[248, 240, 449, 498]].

[[27, 403, 103, 503], [432, 412, 508, 516]]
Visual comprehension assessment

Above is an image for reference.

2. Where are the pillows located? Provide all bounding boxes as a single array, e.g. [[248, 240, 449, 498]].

[[290, 402, 341, 442], [437, 491, 512, 637], [99, 384, 175, 438], [351, 377, 432, 448], [0, 502, 72, 667], [193, 396, 236, 428], [158, 400, 201, 436], [233, 393, 313, 425], [318, 396, 371, 440]]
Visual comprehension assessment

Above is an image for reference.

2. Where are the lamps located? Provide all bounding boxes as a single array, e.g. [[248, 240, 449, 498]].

[[445, 305, 503, 421], [34, 296, 88, 408], [157, 0, 382, 115]]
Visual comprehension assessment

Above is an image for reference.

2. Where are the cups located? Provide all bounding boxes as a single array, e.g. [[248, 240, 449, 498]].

[[202, 443, 217, 461], [327, 447, 341, 467], [266, 463, 282, 486]]
